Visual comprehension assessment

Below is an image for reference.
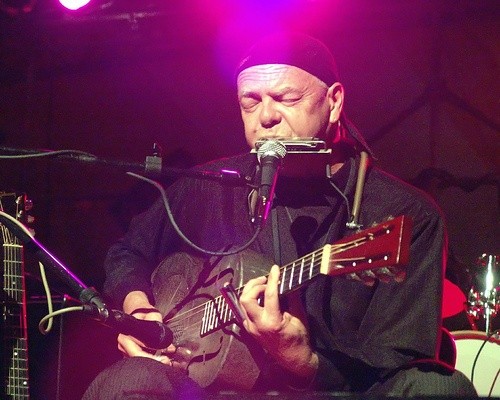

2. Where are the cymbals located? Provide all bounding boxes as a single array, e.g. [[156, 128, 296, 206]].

[[441, 278, 466, 319]]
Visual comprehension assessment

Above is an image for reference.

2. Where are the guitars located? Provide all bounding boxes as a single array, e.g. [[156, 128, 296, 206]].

[[122, 214, 413, 396], [0, 191, 37, 400]]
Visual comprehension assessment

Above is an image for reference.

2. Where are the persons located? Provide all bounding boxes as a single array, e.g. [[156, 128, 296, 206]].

[[81, 32, 479, 400]]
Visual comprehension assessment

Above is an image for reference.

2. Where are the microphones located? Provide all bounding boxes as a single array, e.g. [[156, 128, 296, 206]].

[[81, 304, 174, 350], [254, 140, 287, 230]]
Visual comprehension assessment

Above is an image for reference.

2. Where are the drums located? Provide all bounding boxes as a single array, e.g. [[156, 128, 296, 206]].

[[449, 331, 500, 398], [466, 251, 500, 331]]
